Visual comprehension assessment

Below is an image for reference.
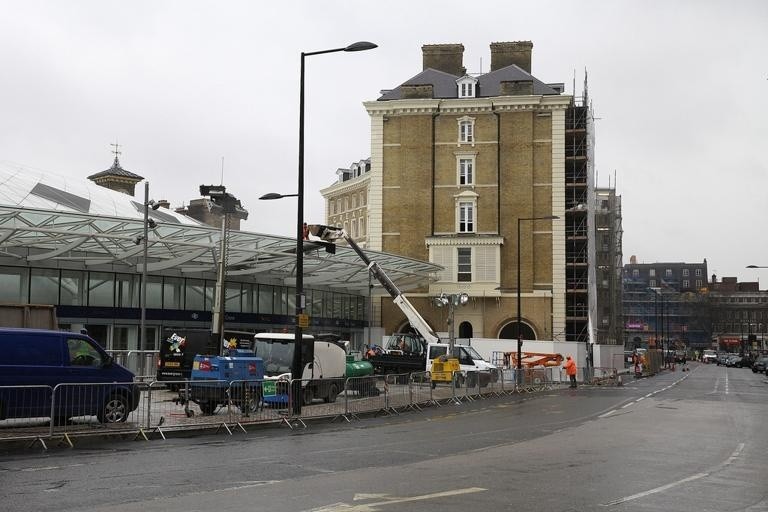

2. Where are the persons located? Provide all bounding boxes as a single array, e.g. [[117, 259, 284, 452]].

[[72, 327, 94, 359], [363, 336, 408, 358], [681, 353, 690, 371], [562, 354, 577, 388]]
